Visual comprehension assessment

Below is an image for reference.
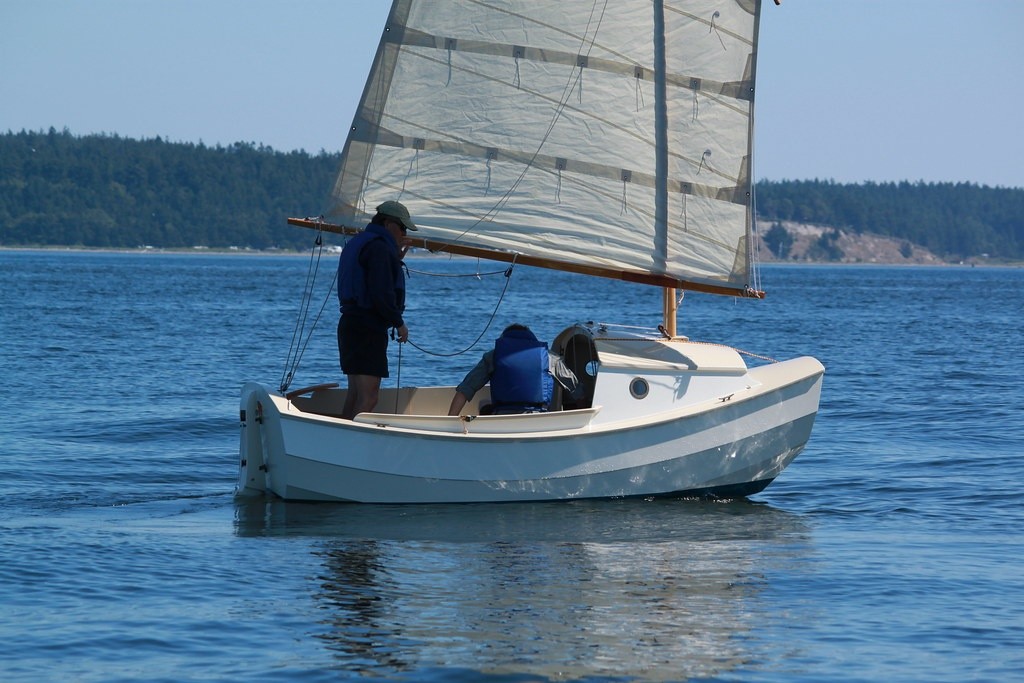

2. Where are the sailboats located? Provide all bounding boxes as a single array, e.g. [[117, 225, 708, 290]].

[[237, 0, 826, 505]]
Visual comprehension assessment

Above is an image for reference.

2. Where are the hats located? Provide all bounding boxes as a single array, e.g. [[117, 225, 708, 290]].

[[376, 201, 418, 231]]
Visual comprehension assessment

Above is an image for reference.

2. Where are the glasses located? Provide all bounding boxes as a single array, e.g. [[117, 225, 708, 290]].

[[392, 221, 407, 231]]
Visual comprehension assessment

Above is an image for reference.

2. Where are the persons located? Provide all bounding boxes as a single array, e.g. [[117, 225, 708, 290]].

[[336, 199, 418, 420], [446, 323, 588, 416]]
